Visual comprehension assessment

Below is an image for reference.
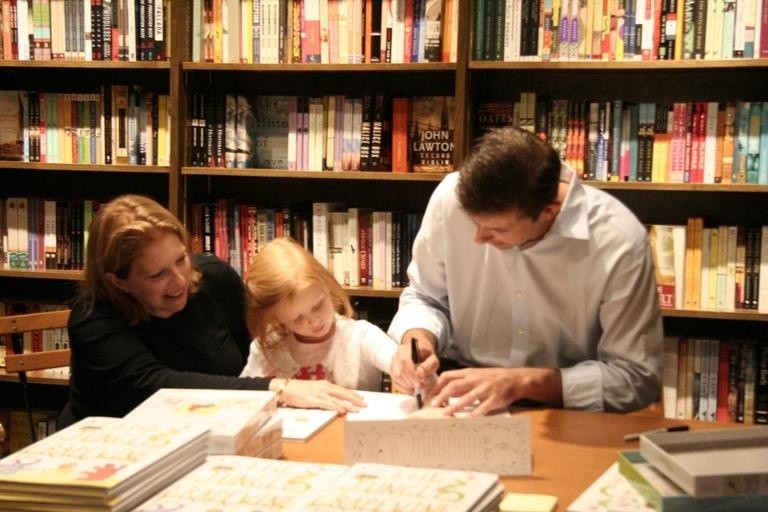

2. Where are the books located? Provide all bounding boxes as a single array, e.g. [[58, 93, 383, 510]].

[[468, 0, 767, 425], [183, 1, 459, 332], [0, 0, 171, 458], [1, 391, 767, 512]]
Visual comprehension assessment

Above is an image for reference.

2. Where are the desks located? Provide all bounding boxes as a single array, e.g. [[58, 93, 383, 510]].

[[277, 407, 759, 512]]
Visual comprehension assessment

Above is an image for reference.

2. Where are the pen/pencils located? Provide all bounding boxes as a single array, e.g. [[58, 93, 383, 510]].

[[624, 426, 689, 441], [411, 337, 423, 411]]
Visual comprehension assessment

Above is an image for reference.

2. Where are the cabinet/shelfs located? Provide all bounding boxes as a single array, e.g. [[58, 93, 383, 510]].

[[0, 1, 768, 387]]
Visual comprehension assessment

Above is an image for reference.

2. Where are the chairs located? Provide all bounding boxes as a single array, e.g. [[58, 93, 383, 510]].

[[1, 308, 72, 440]]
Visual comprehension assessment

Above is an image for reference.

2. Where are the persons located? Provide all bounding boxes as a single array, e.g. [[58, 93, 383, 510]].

[[55, 194, 368, 433], [240, 236, 450, 409], [387, 127, 664, 419]]
